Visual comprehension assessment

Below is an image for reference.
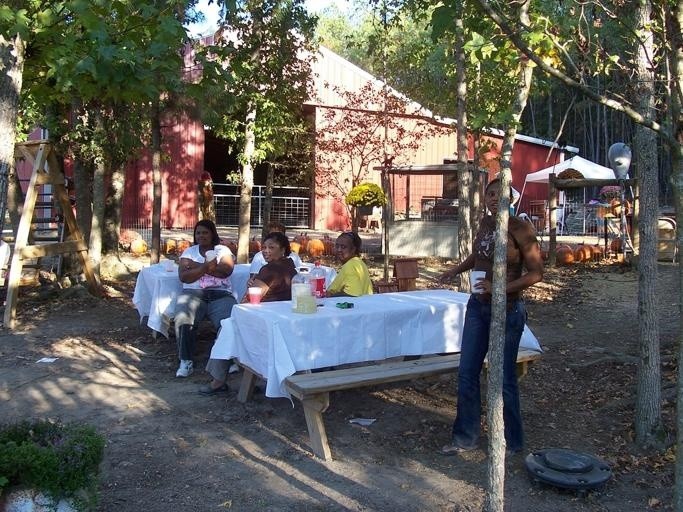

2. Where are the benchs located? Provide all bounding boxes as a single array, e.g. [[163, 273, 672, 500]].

[[286, 345, 542, 465]]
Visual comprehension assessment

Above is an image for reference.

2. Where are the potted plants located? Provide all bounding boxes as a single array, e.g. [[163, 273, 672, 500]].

[[345, 179, 389, 216]]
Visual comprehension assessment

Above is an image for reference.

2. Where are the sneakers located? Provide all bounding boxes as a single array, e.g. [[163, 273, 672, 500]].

[[176, 360, 195, 378], [229, 363, 239, 375]]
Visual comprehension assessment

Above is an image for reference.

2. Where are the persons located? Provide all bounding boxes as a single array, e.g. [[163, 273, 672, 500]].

[[174, 219, 238, 377], [248, 221, 304, 279], [196, 231, 297, 397], [433, 178, 545, 456], [323, 230, 374, 298]]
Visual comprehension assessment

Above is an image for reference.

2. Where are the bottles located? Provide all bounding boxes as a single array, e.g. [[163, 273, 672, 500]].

[[308, 261, 328, 306], [291, 267, 317, 314]]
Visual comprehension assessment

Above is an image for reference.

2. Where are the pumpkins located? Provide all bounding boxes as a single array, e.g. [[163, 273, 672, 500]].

[[131, 240, 148, 254], [541, 197, 630, 264], [164, 232, 334, 258]]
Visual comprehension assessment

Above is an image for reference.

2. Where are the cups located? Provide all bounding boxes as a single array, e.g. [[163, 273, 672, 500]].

[[248, 286, 262, 304], [469, 270, 487, 294], [165, 260, 175, 272]]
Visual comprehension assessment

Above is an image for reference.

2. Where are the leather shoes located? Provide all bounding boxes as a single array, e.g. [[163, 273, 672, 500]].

[[199, 381, 228, 395], [436, 442, 474, 455]]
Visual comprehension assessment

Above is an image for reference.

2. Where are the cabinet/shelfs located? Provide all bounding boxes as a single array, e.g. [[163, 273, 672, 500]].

[[2, 138, 110, 331]]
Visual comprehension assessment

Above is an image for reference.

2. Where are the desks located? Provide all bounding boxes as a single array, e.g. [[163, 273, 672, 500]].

[[131, 260, 340, 337], [211, 287, 541, 404]]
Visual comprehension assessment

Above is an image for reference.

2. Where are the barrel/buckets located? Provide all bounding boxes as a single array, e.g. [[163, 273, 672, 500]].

[[290, 265, 318, 313]]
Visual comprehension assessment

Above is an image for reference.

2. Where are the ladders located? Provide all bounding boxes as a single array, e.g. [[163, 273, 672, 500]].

[[0, 174, 68, 286]]
